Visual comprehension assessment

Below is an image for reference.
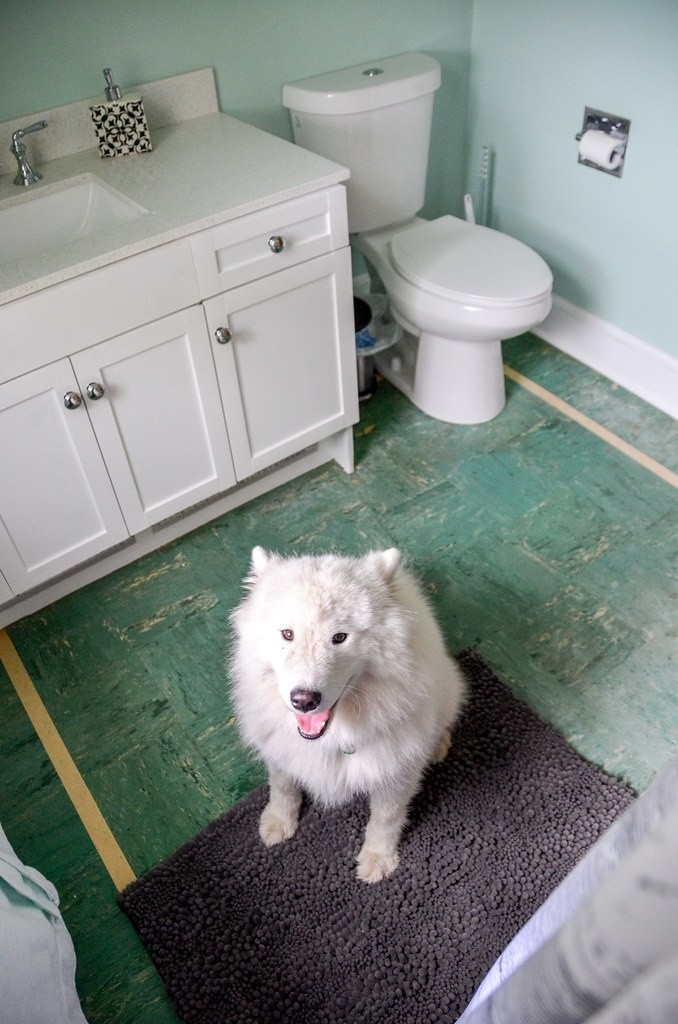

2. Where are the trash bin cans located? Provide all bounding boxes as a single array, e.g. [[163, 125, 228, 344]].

[[354, 296, 378, 404]]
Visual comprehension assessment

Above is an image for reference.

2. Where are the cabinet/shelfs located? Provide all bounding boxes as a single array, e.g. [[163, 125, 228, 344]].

[[0, 182, 360, 597]]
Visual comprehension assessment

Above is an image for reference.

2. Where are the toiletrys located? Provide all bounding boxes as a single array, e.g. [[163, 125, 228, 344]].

[[80, 67, 155, 161]]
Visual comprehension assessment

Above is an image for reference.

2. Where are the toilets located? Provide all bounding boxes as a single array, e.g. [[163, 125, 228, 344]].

[[282, 50, 555, 427]]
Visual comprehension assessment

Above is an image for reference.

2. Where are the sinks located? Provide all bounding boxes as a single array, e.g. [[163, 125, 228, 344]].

[[0, 173, 156, 272]]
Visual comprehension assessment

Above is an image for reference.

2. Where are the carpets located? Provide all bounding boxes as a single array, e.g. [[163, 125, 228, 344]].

[[115, 643, 639, 1024]]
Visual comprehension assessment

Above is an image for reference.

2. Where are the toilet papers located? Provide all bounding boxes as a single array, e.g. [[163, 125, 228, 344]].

[[577, 128, 624, 172]]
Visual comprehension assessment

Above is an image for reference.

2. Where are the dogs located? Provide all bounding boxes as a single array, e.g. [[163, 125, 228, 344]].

[[230, 546, 469, 884]]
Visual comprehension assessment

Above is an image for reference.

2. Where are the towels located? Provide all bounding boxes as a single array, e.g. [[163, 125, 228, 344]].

[[1, 819, 84, 1024]]
